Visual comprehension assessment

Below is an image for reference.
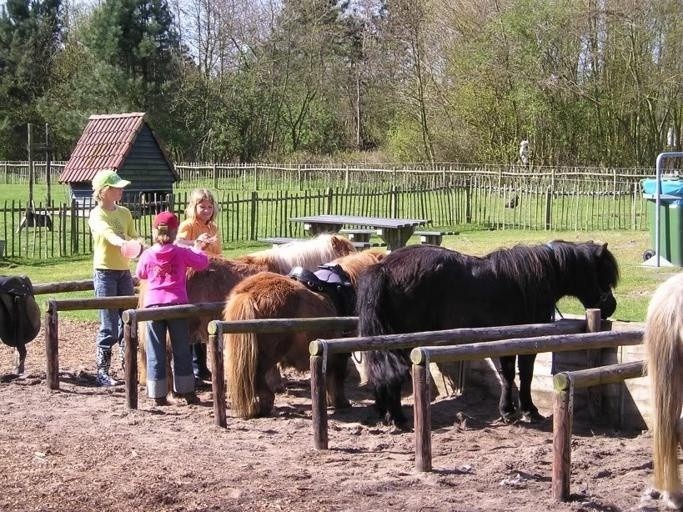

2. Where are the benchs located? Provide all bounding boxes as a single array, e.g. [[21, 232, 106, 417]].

[[341, 227, 459, 247], [259, 236, 387, 253]]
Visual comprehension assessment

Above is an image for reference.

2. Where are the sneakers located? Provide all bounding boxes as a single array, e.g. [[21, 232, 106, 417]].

[[176, 394, 198, 407], [149, 397, 166, 406]]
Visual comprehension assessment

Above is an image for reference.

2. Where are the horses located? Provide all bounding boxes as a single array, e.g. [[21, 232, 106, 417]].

[[134, 233, 361, 385], [362, 237, 614, 436], [222, 244, 391, 419]]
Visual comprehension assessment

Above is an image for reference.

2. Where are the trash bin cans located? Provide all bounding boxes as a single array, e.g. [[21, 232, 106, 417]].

[[639, 177, 683, 269]]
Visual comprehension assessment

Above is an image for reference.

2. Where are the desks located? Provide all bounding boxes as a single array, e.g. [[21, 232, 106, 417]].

[[289, 213, 432, 252]]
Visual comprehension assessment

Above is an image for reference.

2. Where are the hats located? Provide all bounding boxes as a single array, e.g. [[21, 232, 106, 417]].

[[154, 211, 179, 231], [92, 169, 131, 194]]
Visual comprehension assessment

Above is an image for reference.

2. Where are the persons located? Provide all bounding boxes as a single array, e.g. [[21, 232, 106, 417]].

[[136, 211, 208, 407], [88, 169, 144, 387], [176, 189, 222, 387]]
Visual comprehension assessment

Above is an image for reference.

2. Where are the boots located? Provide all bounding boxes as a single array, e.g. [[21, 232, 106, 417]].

[[193, 344, 213, 381], [118, 346, 131, 379], [96, 347, 119, 386]]
